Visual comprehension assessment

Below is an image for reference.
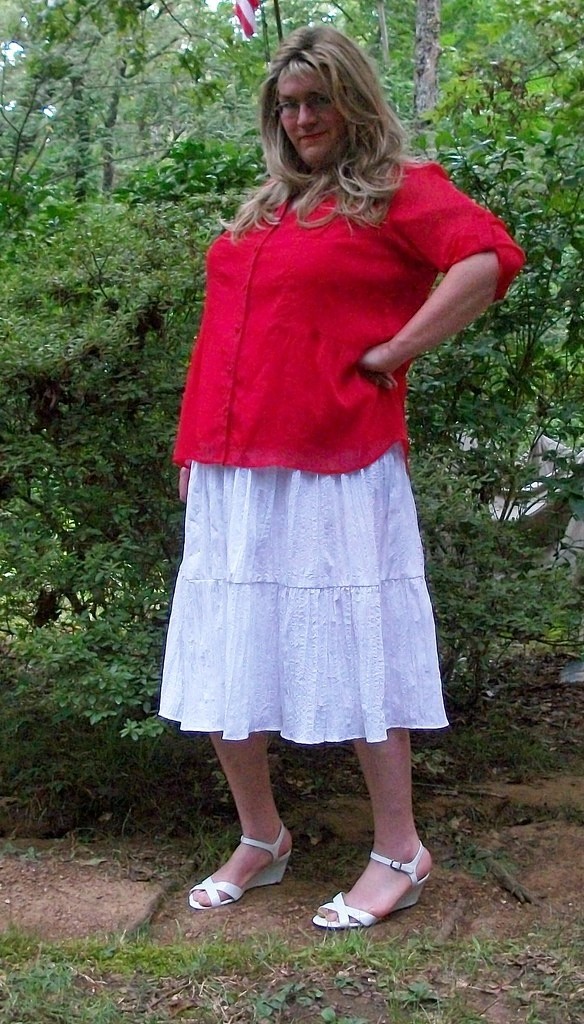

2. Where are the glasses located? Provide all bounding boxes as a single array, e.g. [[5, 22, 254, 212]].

[[274, 92, 335, 116]]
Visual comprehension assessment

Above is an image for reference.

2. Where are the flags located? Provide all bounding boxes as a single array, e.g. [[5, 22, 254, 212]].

[[235, 0, 267, 37]]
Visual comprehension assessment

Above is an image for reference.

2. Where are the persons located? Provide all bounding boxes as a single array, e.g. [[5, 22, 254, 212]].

[[155, 28, 522, 928]]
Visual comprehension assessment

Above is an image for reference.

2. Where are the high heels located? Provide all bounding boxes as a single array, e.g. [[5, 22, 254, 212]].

[[188, 819, 292, 911], [312, 839, 430, 929]]
